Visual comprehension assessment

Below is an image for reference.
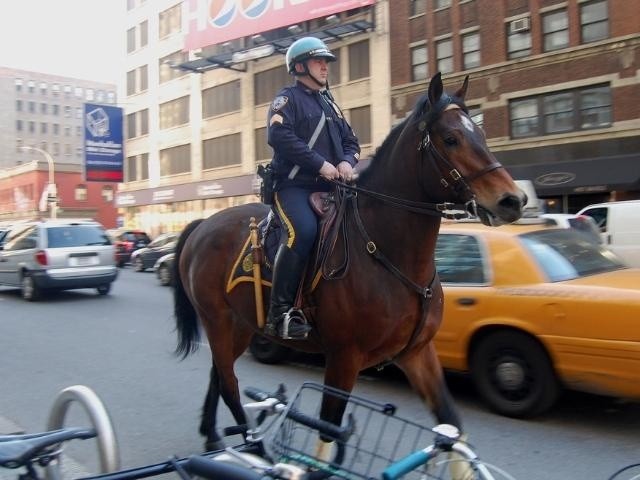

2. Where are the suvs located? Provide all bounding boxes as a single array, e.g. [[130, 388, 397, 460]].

[[0, 219, 118, 301]]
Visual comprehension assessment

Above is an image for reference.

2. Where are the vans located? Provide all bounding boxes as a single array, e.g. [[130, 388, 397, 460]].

[[576, 200, 640, 268]]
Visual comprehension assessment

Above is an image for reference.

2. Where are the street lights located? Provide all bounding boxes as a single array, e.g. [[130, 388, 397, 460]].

[[19, 146, 57, 218]]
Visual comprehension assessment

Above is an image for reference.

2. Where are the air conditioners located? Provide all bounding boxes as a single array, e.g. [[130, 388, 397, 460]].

[[510, 18, 530, 34]]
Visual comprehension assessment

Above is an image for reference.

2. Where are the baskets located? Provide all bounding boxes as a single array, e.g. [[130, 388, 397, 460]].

[[270, 380, 480, 479]]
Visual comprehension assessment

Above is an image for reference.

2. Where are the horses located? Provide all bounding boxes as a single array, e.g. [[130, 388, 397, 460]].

[[170, 70, 529, 480]]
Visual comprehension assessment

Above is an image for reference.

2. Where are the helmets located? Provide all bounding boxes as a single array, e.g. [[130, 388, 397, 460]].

[[286, 37, 336, 75]]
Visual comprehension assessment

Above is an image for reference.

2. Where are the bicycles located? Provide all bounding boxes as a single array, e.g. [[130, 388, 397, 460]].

[[0, 384, 640, 480]]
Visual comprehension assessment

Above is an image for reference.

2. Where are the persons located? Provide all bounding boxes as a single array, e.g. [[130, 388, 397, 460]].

[[266, 36, 361, 338]]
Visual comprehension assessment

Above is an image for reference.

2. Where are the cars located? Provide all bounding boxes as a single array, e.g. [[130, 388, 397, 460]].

[[248, 212, 640, 422], [112, 231, 182, 286]]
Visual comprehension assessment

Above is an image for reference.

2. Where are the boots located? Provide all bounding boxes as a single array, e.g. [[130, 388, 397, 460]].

[[263, 242, 312, 338]]
